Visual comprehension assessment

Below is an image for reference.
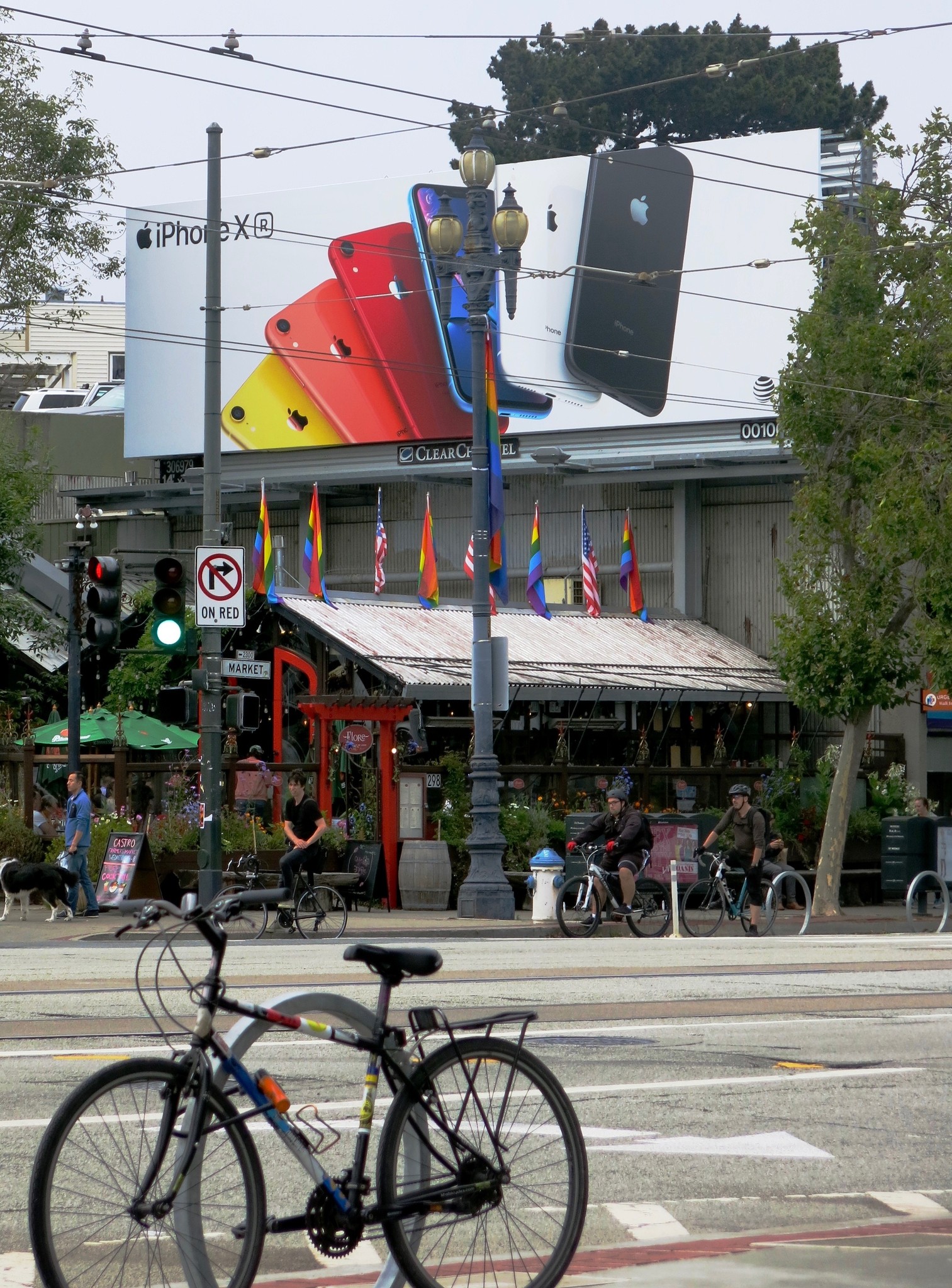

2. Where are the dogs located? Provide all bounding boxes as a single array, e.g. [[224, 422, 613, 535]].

[[0, 856, 81, 924]]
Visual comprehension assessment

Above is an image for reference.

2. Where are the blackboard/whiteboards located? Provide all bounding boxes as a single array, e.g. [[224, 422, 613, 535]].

[[94, 832, 165, 907], [334, 839, 388, 901]]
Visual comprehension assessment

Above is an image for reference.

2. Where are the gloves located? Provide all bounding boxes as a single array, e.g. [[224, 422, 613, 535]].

[[606, 841, 615, 852], [693, 846, 706, 858], [742, 865, 758, 879], [567, 841, 577, 852]]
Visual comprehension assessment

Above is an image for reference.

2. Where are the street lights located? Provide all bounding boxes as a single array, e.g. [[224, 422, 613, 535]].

[[75, 504, 106, 560], [428, 125, 533, 922]]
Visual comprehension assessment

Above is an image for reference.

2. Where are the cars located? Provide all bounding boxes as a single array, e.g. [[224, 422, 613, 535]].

[[11, 380, 125, 416]]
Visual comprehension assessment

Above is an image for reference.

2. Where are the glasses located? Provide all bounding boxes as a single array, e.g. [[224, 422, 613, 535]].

[[732, 795, 743, 799], [607, 800, 620, 807]]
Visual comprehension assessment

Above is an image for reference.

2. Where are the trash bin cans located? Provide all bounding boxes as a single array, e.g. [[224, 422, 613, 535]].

[[882, 816, 937, 891], [565, 812, 720, 897]]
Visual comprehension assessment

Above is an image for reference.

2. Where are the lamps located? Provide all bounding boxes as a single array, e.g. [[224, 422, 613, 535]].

[[529, 447, 596, 469], [180, 467, 256, 491]]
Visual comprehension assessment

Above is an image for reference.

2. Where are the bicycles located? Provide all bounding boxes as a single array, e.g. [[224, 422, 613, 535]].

[[26, 886, 591, 1288], [680, 848, 779, 939], [554, 842, 673, 938], [209, 853, 349, 941]]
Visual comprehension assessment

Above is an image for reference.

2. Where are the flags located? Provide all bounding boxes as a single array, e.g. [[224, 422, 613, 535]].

[[417, 496, 439, 610], [487, 333, 509, 606], [619, 510, 655, 625], [527, 505, 552, 621], [375, 491, 387, 596], [582, 508, 601, 618], [251, 482, 285, 604], [463, 528, 497, 616], [303, 486, 339, 610]]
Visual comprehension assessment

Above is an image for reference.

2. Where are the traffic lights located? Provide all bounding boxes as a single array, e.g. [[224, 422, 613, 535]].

[[88, 560, 129, 652], [226, 691, 261, 732], [151, 557, 189, 651], [157, 685, 198, 728]]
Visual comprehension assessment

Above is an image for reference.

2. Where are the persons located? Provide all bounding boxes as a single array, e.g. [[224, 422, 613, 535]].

[[51, 772, 100, 918], [279, 768, 327, 889], [754, 806, 805, 911], [105, 780, 129, 814], [693, 783, 766, 937], [234, 744, 274, 837], [911, 797, 938, 818], [30, 790, 59, 836], [567, 789, 650, 926], [100, 776, 112, 796]]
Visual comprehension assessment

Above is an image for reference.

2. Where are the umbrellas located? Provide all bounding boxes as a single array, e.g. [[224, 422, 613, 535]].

[[13, 702, 192, 818]]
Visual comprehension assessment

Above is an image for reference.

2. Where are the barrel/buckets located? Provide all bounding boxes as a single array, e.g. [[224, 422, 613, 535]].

[[398, 839, 452, 911]]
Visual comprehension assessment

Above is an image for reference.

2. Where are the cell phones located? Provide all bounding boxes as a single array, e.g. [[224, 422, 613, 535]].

[[215, 144, 694, 449]]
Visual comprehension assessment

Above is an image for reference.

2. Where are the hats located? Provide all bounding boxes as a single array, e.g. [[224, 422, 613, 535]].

[[249, 745, 264, 754]]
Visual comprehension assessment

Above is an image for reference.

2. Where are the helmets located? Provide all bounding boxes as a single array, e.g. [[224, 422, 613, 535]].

[[729, 783, 751, 797], [606, 789, 629, 807]]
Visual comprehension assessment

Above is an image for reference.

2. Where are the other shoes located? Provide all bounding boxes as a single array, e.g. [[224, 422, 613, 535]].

[[579, 913, 603, 927], [613, 903, 632, 916], [74, 909, 99, 918], [786, 902, 805, 910], [710, 893, 731, 910], [278, 898, 295, 909], [265, 920, 291, 933], [902, 898, 916, 908], [745, 924, 759, 937], [56, 909, 67, 919], [933, 899, 943, 909], [777, 903, 785, 910]]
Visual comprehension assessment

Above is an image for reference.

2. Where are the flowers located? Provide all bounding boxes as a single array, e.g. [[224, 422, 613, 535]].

[[397, 741, 422, 761]]
